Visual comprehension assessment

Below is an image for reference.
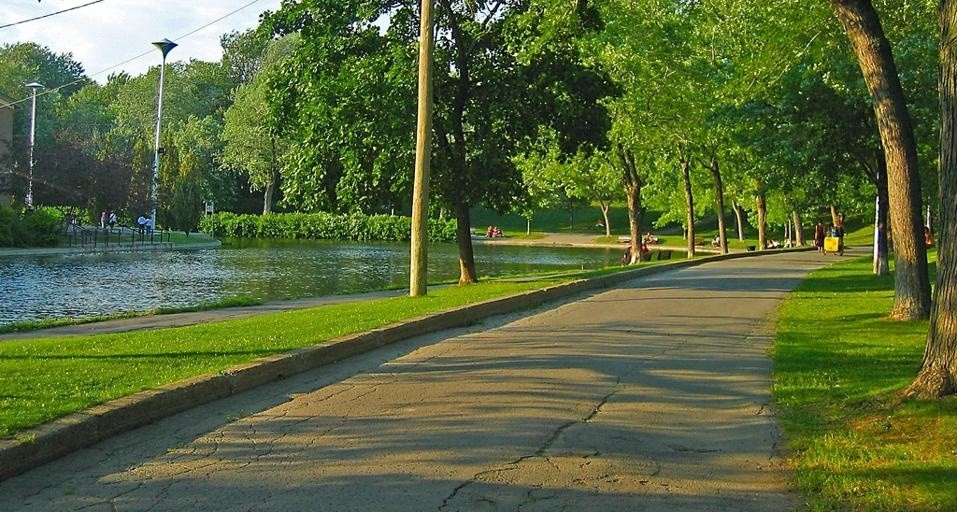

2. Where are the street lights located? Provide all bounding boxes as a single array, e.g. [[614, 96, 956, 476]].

[[150, 37, 178, 230], [25, 82, 43, 210]]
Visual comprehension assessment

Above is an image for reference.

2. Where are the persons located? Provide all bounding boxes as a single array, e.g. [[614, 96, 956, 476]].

[[766, 240, 773, 248], [64, 209, 77, 225], [136, 214, 146, 234], [109, 210, 117, 233], [712, 231, 720, 249], [143, 216, 153, 235], [770, 239, 777, 248], [923, 226, 934, 249], [486, 223, 500, 239], [640, 232, 656, 253], [814, 220, 826, 256]]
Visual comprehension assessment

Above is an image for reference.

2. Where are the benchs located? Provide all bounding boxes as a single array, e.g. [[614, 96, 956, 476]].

[[658, 251, 671, 260], [488, 230, 503, 238], [643, 237, 659, 245], [622, 253, 652, 263]]
